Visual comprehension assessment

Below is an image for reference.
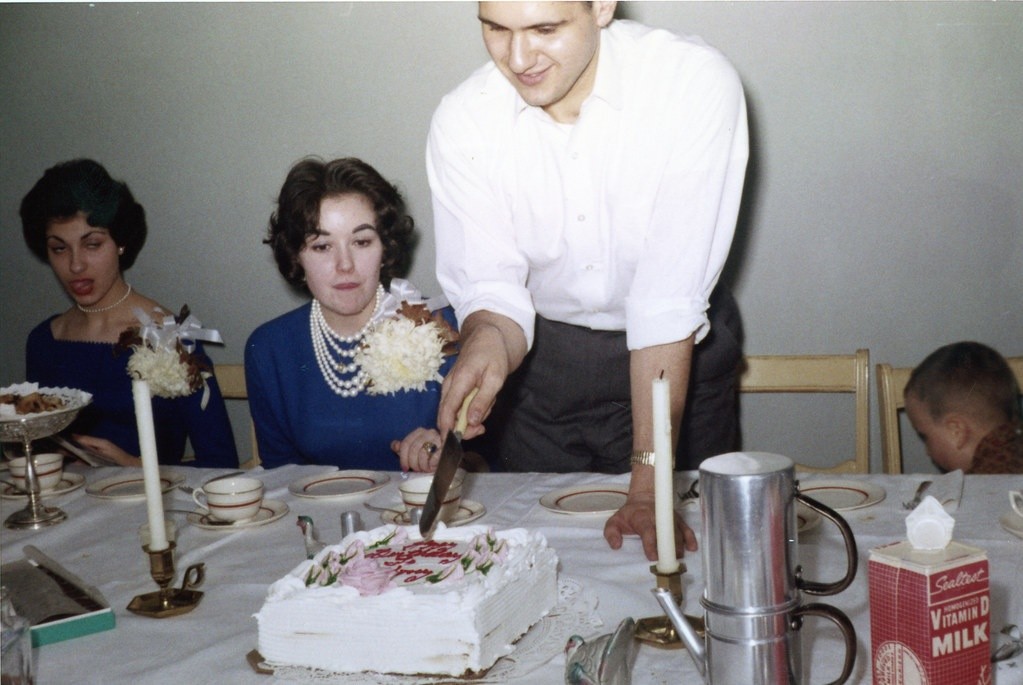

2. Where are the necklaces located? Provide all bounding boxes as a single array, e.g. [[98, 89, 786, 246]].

[[309, 283, 386, 395], [77, 282, 132, 312]]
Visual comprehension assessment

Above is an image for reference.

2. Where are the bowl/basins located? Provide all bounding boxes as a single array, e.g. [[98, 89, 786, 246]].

[[7, 453, 63, 493]]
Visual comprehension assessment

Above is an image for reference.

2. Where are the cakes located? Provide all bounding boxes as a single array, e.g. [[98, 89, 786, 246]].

[[253, 520, 561, 679]]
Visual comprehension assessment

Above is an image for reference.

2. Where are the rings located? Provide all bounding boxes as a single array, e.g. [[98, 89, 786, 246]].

[[423, 442, 438, 455]]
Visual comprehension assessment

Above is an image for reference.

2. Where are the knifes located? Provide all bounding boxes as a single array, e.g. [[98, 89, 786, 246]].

[[419, 387, 481, 539]]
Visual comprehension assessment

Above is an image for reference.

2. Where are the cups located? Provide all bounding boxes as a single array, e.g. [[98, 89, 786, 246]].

[[397, 476, 464, 524], [697, 451, 858, 614], [193, 477, 265, 523]]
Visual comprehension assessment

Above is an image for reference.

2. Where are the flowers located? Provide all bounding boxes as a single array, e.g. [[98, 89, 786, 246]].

[[126, 305, 224, 411], [351, 319, 446, 397]]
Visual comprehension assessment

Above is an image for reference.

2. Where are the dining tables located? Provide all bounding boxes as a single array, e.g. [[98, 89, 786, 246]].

[[1, 461, 1022, 684]]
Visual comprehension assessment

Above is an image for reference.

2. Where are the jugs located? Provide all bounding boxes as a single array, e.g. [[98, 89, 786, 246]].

[[651, 587, 857, 685]]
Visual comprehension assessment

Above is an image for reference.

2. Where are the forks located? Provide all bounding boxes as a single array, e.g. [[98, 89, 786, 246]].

[[902, 480, 931, 510]]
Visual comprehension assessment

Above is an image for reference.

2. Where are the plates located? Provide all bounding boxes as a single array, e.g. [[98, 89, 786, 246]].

[[186, 498, 290, 530], [794, 478, 886, 535], [288, 470, 391, 501], [86, 473, 186, 500], [0, 472, 86, 499], [382, 500, 486, 527], [540, 484, 629, 515], [999, 509, 1023, 539]]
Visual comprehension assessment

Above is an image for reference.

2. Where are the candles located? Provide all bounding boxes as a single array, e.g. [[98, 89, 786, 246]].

[[653, 370, 681, 572], [131, 370, 167, 548]]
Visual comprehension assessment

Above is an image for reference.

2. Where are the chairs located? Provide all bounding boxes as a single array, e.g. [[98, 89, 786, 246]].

[[736, 347, 867, 475], [877, 358, 1023, 475], [215, 363, 262, 469]]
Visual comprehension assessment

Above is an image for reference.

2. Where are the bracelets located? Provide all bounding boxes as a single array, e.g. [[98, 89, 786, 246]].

[[630, 450, 676, 471]]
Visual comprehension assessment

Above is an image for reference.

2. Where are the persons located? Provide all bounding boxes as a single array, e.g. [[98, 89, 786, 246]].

[[245, 154, 464, 472], [902, 341, 1023, 474], [17, 156, 238, 468], [426, 3, 753, 566]]
